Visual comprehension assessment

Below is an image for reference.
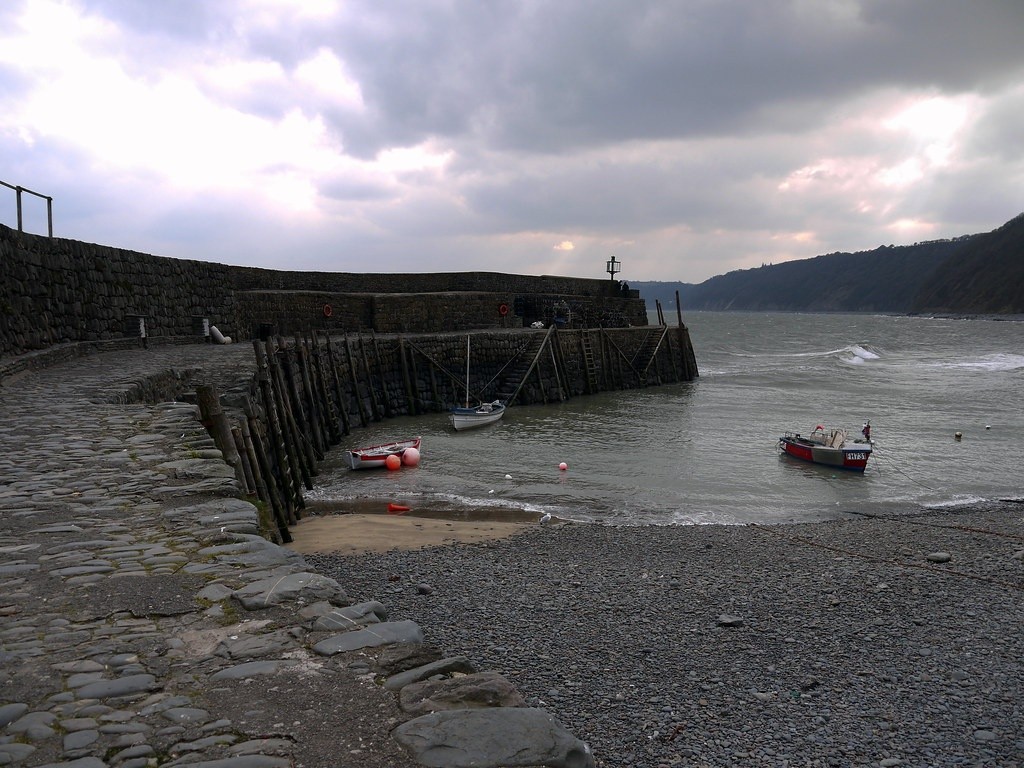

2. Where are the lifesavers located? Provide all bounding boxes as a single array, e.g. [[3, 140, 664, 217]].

[[813, 425, 826, 436], [500, 305, 508, 315]]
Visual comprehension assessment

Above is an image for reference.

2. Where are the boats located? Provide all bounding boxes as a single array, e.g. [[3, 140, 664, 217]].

[[342, 436, 421, 469], [449, 401, 505, 432], [779, 419, 873, 472]]
